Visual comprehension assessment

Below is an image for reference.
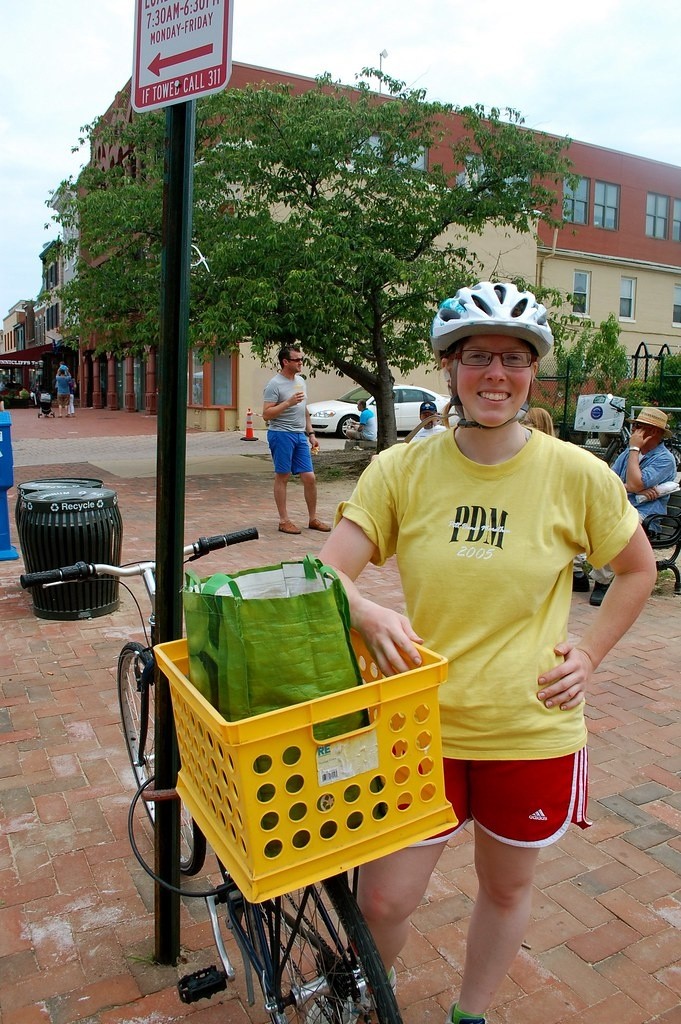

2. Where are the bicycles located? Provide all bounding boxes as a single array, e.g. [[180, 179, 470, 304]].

[[18, 524, 405, 1023]]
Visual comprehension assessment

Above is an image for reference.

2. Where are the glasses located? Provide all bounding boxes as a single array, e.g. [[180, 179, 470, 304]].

[[449, 349, 537, 368], [632, 422, 650, 429], [421, 406, 435, 411], [289, 357, 303, 362]]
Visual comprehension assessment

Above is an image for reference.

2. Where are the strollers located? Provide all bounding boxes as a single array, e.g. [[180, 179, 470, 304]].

[[37, 390, 56, 417]]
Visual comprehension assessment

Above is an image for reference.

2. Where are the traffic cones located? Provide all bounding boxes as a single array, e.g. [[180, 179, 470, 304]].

[[239, 408, 259, 441]]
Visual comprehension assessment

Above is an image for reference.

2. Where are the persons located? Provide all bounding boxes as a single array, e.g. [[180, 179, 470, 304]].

[[345, 399, 377, 441], [408, 401, 446, 443], [0, 376, 39, 406], [571, 406, 676, 606], [261, 346, 331, 534], [518, 407, 554, 436], [306, 278, 659, 1024], [55, 361, 76, 418]]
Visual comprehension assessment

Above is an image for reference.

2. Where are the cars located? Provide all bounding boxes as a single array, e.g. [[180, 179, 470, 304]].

[[303, 383, 463, 439]]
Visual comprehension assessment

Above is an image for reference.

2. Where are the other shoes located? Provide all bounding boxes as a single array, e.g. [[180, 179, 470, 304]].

[[66, 415, 72, 418], [590, 581, 610, 606], [573, 574, 590, 593], [57, 416, 62, 418], [279, 519, 301, 534], [309, 519, 332, 532]]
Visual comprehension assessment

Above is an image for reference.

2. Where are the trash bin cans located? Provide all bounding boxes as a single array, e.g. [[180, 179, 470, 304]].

[[19, 488, 124, 620], [15, 478, 104, 595]]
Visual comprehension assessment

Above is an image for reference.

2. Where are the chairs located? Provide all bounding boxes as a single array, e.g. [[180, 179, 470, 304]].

[[602, 438, 681, 596]]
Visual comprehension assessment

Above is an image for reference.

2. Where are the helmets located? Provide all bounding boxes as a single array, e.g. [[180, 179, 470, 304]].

[[431, 281, 554, 365]]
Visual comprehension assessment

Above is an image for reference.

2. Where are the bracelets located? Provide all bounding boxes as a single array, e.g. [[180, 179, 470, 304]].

[[629, 446, 640, 451], [308, 432, 315, 435]]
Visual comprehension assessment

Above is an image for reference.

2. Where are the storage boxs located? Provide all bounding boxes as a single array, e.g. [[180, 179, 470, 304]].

[[152, 627, 457, 903]]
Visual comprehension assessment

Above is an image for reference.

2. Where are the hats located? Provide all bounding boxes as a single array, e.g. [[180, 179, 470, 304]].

[[420, 402, 437, 414], [626, 407, 673, 438]]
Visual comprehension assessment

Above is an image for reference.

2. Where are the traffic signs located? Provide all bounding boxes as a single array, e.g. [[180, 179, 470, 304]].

[[131, 1, 235, 113]]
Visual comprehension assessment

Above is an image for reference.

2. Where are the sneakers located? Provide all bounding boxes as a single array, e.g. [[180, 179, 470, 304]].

[[306, 966, 398, 1023], [446, 1002, 485, 1024]]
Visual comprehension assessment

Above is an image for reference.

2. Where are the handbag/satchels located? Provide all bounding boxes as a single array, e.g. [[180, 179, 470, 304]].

[[183, 551, 387, 856]]
